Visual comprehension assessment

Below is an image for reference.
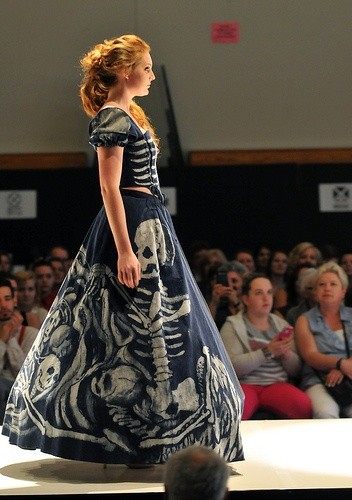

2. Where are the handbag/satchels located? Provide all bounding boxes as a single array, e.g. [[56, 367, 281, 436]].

[[326, 375, 352, 407]]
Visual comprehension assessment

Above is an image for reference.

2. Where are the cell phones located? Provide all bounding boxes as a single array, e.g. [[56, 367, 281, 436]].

[[278, 325, 292, 343], [217, 270, 228, 289]]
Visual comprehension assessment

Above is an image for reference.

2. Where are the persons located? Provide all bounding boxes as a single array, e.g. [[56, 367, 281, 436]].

[[163, 445, 229, 500], [1, 243, 352, 418], [1, 36, 246, 468]]
